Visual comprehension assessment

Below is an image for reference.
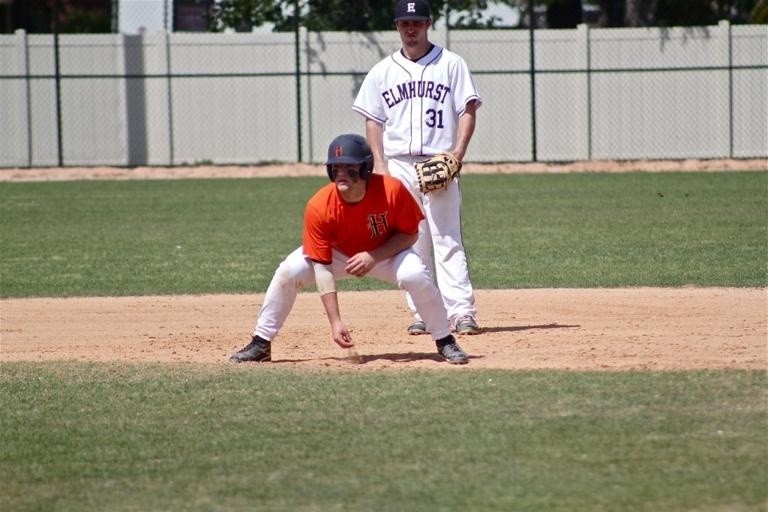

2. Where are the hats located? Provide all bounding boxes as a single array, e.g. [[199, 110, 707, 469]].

[[392, 0, 430, 22]]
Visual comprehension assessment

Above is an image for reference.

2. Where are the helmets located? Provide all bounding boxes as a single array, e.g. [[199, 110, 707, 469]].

[[324, 134, 372, 165]]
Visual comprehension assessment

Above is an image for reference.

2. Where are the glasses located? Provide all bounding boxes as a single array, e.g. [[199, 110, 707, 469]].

[[331, 169, 358, 178]]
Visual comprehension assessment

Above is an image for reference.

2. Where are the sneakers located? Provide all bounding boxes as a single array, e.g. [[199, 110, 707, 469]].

[[436, 335, 468, 364], [407, 320, 427, 335], [456, 315, 479, 335], [229, 335, 271, 363]]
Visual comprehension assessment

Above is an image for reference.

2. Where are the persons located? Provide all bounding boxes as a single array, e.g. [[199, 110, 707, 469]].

[[351, 0, 483, 336], [229, 134, 470, 364]]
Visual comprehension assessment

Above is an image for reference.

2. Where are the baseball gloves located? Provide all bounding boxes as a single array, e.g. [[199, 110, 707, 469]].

[[413, 151, 462, 195]]
